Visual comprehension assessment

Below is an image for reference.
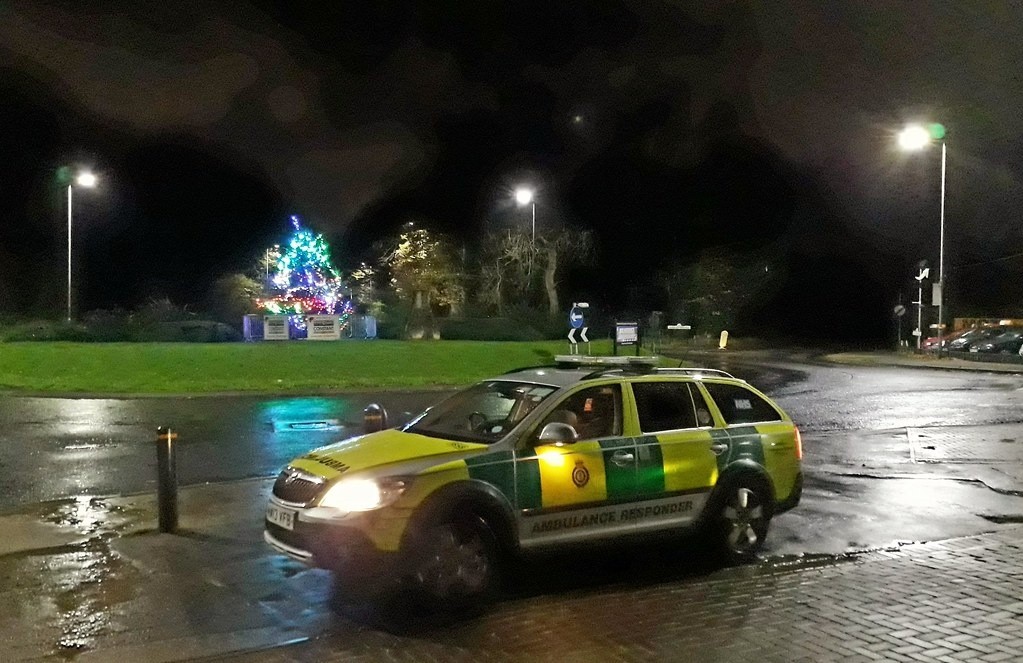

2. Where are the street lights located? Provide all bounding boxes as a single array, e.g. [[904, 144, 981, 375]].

[[895, 124, 944, 359], [67, 168, 97, 325], [513, 187, 537, 256]]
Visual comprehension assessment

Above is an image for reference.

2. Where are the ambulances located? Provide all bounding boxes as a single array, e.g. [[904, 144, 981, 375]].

[[261, 354, 803, 612]]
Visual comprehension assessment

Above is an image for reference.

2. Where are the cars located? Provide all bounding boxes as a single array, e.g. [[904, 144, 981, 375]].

[[922, 321, 1022, 357]]
